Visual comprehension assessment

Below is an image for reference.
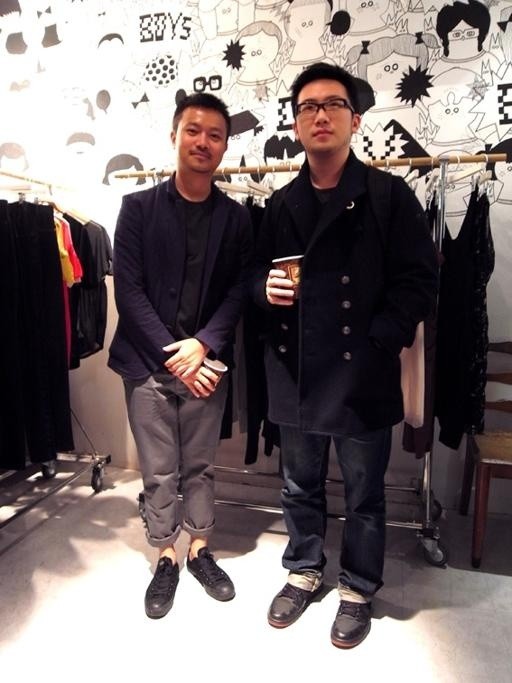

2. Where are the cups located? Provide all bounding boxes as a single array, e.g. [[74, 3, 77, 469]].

[[272, 254, 302, 300]]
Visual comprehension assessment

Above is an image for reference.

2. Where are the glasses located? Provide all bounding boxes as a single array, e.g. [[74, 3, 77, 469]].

[[294, 98, 355, 115], [193, 75, 222, 92], [447, 28, 480, 42]]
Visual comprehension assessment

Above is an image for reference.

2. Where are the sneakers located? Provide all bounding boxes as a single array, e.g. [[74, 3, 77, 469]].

[[144, 555, 179, 619], [186, 546, 236, 601]]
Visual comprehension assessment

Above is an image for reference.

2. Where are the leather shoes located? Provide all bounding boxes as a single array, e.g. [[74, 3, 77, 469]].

[[268, 582, 324, 629], [331, 598, 375, 649]]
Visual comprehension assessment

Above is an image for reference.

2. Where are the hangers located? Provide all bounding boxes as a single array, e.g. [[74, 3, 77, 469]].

[[149, 152, 491, 209], [5, 174, 95, 228]]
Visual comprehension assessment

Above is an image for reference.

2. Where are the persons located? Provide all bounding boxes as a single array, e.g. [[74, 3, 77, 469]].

[[242, 61, 441, 651], [104, 90, 257, 619]]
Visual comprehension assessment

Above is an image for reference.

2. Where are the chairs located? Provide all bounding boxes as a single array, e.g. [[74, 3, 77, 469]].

[[457, 340, 512, 568]]
